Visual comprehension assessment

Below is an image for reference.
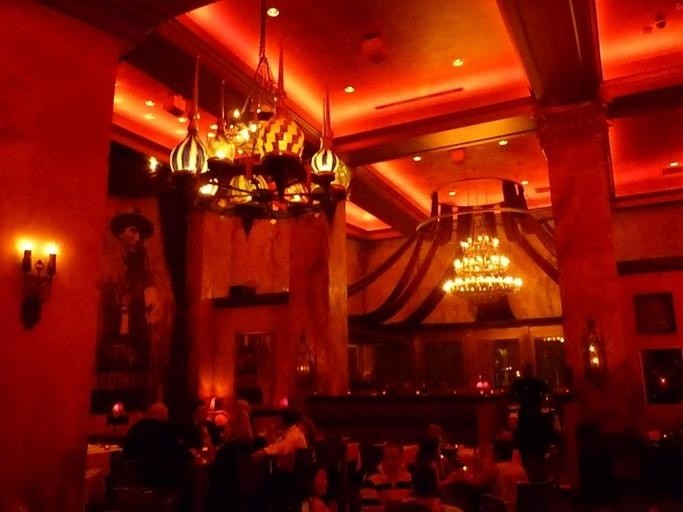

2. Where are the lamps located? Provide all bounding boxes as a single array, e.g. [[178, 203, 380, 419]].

[[444, 181, 523, 305], [169, 0, 352, 239], [21, 241, 55, 330]]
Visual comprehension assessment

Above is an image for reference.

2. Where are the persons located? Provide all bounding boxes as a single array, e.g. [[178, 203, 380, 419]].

[[97, 207, 164, 373]]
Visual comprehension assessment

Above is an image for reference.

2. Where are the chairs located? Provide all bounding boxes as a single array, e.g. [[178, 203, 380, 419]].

[[108, 430, 577, 511]]
